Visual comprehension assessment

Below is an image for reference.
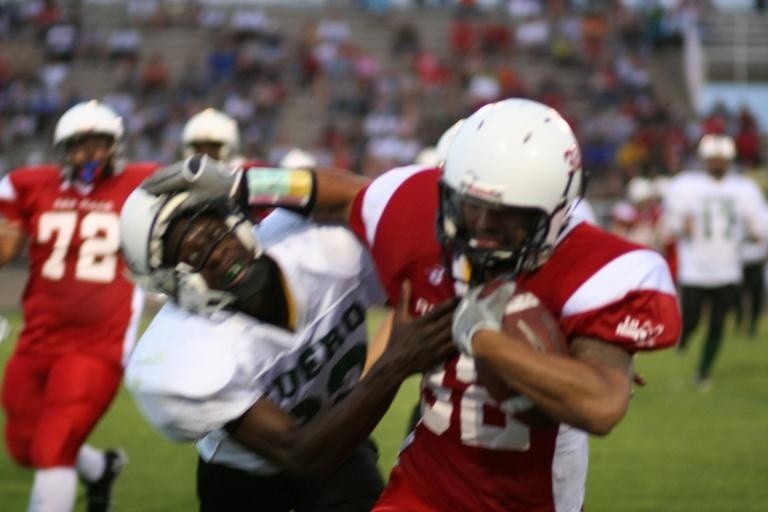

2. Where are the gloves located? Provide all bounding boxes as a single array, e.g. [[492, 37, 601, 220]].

[[451, 283, 517, 357], [142, 153, 245, 213]]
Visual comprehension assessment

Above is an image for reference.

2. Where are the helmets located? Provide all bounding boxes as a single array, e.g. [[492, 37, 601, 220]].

[[438, 97, 584, 271], [180, 108, 239, 160], [697, 133, 737, 160], [53, 99, 124, 150], [117, 180, 259, 317]]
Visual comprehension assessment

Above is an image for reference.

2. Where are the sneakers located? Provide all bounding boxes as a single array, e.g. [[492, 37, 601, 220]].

[[87, 448, 127, 512]]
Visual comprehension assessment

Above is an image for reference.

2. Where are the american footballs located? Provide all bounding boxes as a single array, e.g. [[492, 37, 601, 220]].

[[478, 281, 571, 427]]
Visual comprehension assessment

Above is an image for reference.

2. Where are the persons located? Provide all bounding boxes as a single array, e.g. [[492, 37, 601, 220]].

[[610, 133, 768, 392], [1, 1, 768, 199], [0, 99, 681, 512]]
